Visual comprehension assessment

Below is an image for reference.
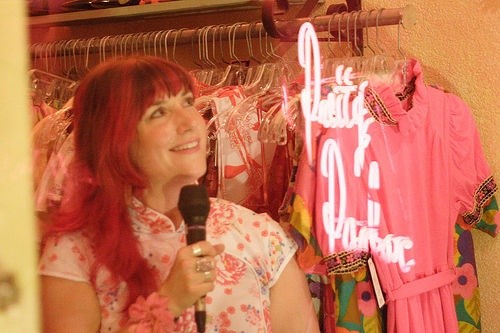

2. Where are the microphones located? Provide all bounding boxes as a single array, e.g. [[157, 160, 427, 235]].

[[177, 184, 211, 333]]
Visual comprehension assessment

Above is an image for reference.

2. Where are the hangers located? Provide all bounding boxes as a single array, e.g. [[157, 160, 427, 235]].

[[26, 7, 466, 163]]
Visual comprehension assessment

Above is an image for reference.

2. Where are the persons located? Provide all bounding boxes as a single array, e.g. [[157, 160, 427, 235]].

[[31, 55, 322, 333]]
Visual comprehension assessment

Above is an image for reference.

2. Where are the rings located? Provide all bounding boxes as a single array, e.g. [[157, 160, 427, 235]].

[[195, 257, 211, 273], [192, 242, 201, 256], [204, 270, 212, 283]]
